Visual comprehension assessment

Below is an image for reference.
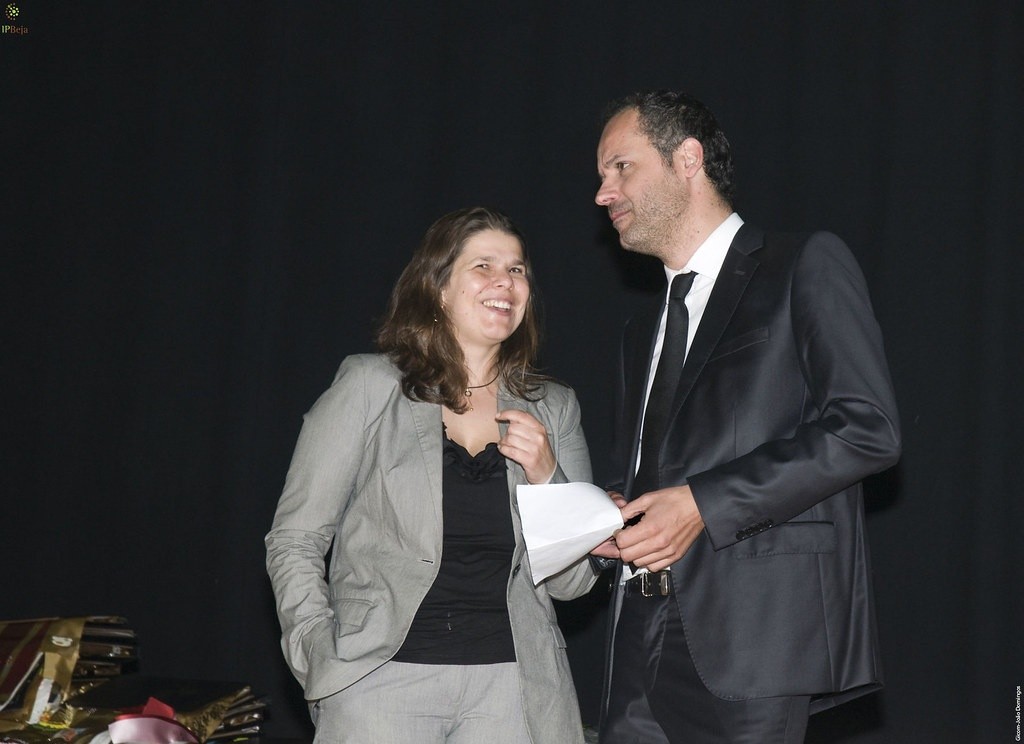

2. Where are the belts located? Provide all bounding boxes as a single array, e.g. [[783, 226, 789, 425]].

[[624, 570, 675, 599]]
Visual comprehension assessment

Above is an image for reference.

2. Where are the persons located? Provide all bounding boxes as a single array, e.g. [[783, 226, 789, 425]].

[[264, 206, 601, 744], [587, 88, 904, 744]]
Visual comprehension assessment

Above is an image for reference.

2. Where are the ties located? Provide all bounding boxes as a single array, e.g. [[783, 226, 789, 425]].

[[627, 270, 699, 526]]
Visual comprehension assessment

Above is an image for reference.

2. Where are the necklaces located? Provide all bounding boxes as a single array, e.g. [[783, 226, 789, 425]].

[[465, 368, 500, 411]]
